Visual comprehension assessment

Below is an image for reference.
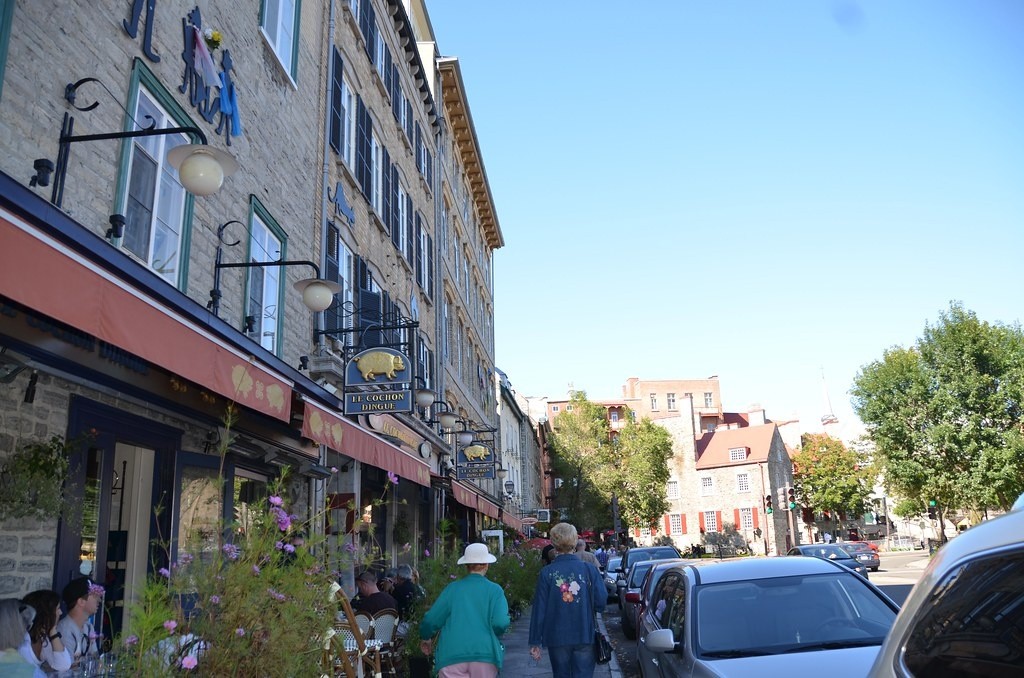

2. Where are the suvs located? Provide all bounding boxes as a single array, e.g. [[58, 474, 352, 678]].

[[615, 545, 683, 611]]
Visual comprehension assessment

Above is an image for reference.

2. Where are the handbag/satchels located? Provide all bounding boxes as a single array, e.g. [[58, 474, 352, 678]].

[[595, 630, 614, 665]]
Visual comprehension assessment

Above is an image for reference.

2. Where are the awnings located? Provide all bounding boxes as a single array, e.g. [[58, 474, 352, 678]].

[[0, 205, 294, 423], [296, 392, 431, 487], [435, 473, 522, 532]]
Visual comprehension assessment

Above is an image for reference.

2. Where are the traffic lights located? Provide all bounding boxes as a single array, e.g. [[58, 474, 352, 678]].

[[777, 487, 789, 512], [928, 500, 936, 519], [764, 495, 772, 515], [787, 487, 796, 509]]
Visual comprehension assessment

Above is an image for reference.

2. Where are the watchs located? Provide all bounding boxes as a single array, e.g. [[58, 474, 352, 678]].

[[50, 632, 62, 641]]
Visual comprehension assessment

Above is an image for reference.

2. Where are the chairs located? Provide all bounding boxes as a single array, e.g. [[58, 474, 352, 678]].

[[332, 608, 400, 678], [776, 593, 828, 642]]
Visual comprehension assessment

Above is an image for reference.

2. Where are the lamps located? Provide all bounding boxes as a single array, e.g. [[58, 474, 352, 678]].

[[496, 460, 508, 478], [206, 289, 222, 309], [203, 425, 221, 452], [415, 376, 475, 446], [106, 214, 126, 239], [242, 315, 255, 333], [29, 159, 54, 187], [206, 220, 341, 319], [298, 355, 309, 370], [23, 367, 38, 403], [52, 78, 242, 217]]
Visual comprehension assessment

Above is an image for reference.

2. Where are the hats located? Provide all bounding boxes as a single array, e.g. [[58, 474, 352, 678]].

[[457, 543, 497, 564], [62, 577, 107, 605]]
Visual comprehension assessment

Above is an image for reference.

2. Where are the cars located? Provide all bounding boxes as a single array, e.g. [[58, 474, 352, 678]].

[[785, 545, 869, 581], [829, 541, 880, 572], [865, 494, 1024, 678], [913, 540, 924, 550], [600, 546, 903, 678]]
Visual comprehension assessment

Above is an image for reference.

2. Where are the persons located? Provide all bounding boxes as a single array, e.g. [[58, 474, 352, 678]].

[[281, 531, 426, 635], [529, 523, 628, 678], [0, 577, 101, 678], [691, 544, 702, 558], [417, 543, 512, 678]]
